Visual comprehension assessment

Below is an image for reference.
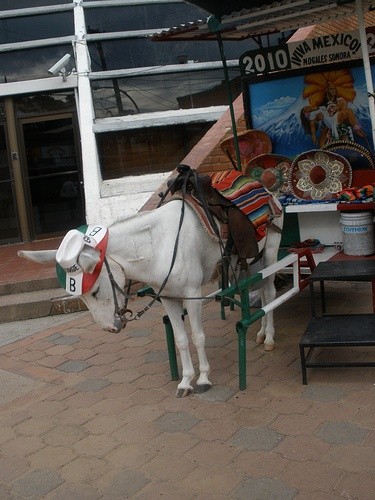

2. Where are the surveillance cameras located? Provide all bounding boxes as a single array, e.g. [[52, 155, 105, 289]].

[[47, 53, 72, 76]]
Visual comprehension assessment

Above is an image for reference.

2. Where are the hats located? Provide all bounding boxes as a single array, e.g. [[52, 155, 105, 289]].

[[55, 223, 109, 296]]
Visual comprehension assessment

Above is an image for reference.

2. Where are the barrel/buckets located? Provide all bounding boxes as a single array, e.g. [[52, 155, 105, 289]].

[[338, 212, 375, 257]]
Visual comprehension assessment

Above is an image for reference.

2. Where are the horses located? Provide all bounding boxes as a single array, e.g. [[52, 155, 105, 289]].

[[17, 179, 284, 397]]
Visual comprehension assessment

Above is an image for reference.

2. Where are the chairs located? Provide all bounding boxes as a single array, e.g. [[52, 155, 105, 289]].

[[298, 260, 375, 386]]
